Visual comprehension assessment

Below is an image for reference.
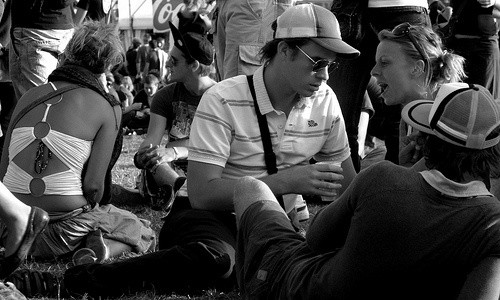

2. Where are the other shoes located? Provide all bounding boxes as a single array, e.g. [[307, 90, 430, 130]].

[[4, 269, 64, 298], [85, 229, 108, 264], [150, 177, 186, 212], [0, 206, 49, 281]]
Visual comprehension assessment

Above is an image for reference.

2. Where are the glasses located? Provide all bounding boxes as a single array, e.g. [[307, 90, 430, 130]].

[[171, 56, 185, 66], [296, 45, 340, 76], [391, 22, 438, 47]]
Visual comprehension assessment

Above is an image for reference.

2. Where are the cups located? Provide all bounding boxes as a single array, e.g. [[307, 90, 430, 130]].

[[315, 162, 342, 202]]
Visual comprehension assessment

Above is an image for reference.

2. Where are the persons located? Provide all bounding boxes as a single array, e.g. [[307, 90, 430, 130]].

[[4, 3, 358, 299], [234, 83, 500, 300], [0, 0, 500, 300]]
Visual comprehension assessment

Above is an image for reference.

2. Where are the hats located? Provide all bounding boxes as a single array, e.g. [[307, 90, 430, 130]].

[[169, 21, 213, 65], [273, 3, 361, 56], [401, 81, 500, 151]]
